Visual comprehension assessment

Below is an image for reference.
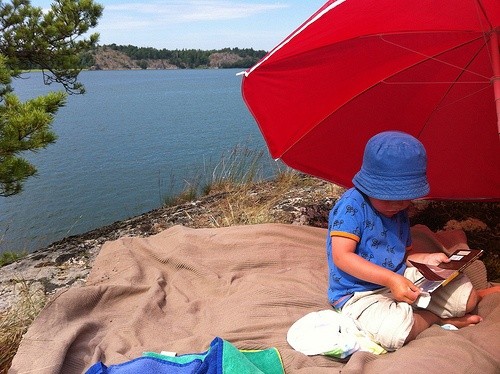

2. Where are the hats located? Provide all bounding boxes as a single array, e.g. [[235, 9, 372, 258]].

[[352, 131, 430, 200]]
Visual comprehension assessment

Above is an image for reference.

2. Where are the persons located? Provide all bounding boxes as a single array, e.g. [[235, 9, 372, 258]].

[[327, 130, 483, 352]]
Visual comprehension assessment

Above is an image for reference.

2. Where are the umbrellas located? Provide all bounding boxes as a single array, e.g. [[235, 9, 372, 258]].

[[236, 0, 500, 203]]
